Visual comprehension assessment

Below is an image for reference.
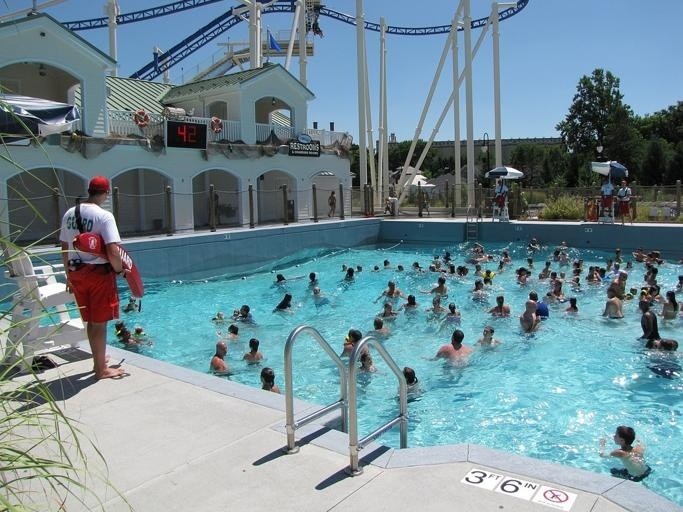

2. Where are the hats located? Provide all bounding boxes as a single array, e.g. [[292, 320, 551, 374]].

[[88, 176, 110, 194]]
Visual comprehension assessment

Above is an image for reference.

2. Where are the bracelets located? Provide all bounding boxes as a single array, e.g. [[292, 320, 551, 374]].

[[114, 270, 123, 275]]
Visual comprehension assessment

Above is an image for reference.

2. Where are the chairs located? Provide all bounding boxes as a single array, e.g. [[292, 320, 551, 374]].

[[0, 236, 93, 377], [490, 190, 511, 221]]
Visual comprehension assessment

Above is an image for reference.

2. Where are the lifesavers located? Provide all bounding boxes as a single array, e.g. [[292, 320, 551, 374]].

[[482, 272, 495, 279], [215, 318, 236, 322], [211, 119, 223, 133], [133, 110, 149, 128]]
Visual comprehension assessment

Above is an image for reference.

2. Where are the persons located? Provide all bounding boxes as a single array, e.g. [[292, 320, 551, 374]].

[[209, 180, 682, 403], [59, 176, 131, 379], [115, 297, 154, 353], [599, 425, 652, 481]]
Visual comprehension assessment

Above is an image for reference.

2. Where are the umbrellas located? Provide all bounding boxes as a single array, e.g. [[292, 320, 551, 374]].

[[591, 160, 629, 184], [396, 166, 437, 187], [485, 166, 523, 188]]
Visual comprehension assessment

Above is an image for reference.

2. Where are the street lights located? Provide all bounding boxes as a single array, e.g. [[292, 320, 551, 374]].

[[480, 131, 491, 208], [596, 129, 604, 186]]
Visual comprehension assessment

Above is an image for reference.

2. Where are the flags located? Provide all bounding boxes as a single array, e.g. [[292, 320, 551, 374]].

[[267, 28, 283, 52]]
